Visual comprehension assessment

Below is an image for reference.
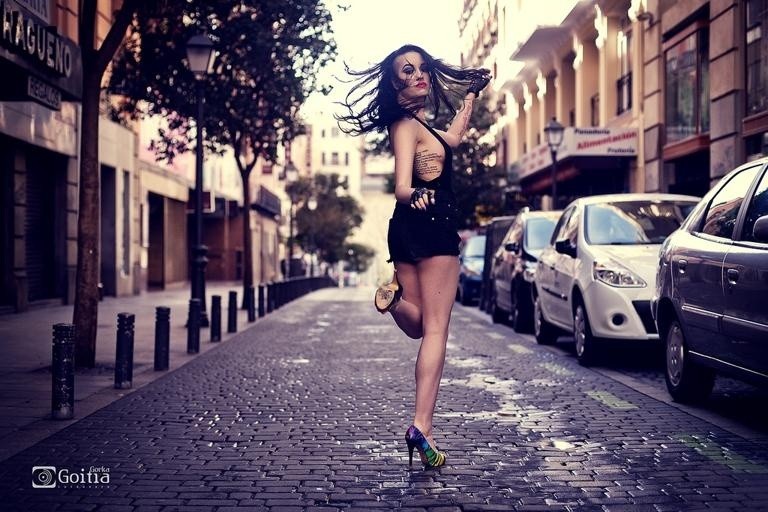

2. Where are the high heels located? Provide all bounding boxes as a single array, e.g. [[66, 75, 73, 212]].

[[374, 269, 403, 313], [405, 425, 446, 468]]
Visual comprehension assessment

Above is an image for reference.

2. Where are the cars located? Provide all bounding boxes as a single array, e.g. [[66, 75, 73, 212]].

[[489, 206, 565, 334], [455, 235, 487, 306], [531, 194, 703, 365], [481, 217, 516, 315], [654, 157, 768, 407]]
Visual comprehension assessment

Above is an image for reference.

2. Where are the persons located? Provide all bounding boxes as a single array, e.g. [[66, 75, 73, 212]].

[[330, 41, 495, 468]]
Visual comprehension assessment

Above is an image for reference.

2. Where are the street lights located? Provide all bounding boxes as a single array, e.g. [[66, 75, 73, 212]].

[[544, 116, 565, 211], [184, 24, 221, 330], [286, 190, 318, 291]]
